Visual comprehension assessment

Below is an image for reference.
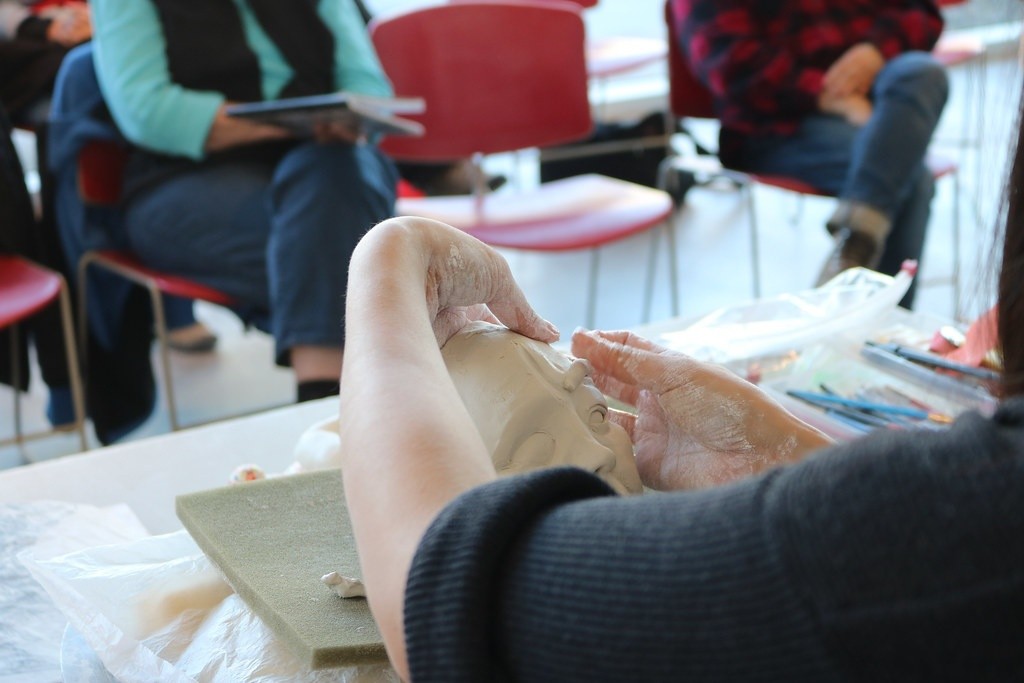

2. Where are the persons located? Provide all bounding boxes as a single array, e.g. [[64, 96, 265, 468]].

[[441, 318, 643, 497], [340, 90, 1024, 683], [0, 0, 394, 435], [673, 0, 951, 310]]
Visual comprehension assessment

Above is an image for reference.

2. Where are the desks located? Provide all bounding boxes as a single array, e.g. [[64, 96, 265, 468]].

[[0, 293, 1001, 683]]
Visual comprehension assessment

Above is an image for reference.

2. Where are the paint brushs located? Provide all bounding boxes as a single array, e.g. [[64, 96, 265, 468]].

[[785, 379, 954, 435]]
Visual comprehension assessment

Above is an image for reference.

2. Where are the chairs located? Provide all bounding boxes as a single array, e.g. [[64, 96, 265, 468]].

[[0, 256, 87, 467], [50, 41, 243, 448], [662, 0, 962, 326], [366, 0, 674, 332]]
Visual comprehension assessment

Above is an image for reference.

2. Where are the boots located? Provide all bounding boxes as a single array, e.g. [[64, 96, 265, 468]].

[[815, 223, 885, 287]]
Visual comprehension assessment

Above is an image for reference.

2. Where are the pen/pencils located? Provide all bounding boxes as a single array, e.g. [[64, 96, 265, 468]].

[[864, 337, 1003, 384]]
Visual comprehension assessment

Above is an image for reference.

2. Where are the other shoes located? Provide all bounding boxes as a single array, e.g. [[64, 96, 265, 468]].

[[423, 157, 507, 195], [151, 319, 218, 352], [46, 379, 91, 431]]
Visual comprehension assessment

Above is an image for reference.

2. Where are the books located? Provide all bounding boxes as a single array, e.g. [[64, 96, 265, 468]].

[[226, 92, 427, 141]]
